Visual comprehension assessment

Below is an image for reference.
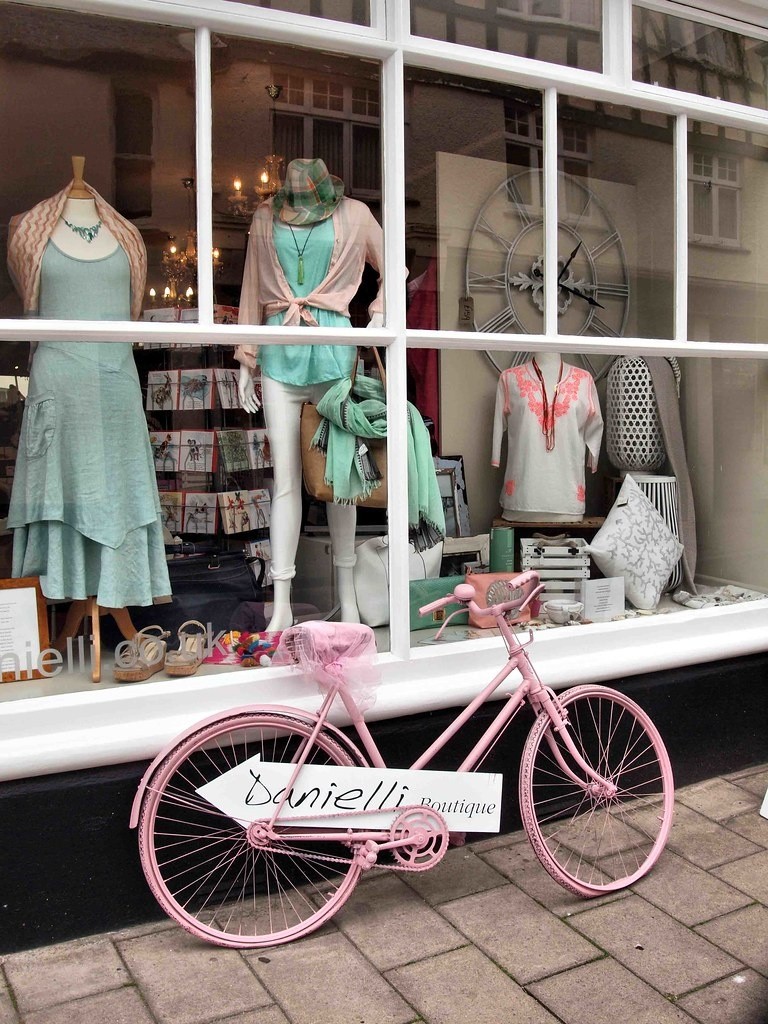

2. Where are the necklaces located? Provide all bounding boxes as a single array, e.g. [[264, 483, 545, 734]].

[[60, 214, 102, 242], [532, 358, 563, 451], [288, 223, 316, 285]]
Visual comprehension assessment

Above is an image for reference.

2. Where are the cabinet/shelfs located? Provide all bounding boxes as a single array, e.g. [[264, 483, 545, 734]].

[[119, 305, 272, 582]]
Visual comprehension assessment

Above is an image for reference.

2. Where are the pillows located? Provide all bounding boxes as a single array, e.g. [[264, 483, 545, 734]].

[[584, 474, 685, 612]]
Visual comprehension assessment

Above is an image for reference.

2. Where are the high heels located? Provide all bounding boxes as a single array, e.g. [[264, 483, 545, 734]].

[[113, 626, 171, 681], [164, 620, 206, 676]]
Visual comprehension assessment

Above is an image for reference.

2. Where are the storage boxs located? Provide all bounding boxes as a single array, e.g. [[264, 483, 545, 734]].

[[295, 537, 377, 627]]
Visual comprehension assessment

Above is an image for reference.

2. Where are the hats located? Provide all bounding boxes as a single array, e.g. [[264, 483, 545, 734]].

[[271, 158, 345, 225]]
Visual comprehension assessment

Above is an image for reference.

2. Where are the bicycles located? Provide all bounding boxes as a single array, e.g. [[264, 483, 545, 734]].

[[128, 570, 676, 949]]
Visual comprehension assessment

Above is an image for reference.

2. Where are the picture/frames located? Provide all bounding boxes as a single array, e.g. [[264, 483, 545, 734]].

[[432, 455, 470, 539], [440, 534, 490, 579]]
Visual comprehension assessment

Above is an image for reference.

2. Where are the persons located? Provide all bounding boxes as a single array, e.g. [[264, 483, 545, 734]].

[[491, 353, 605, 522], [233, 158, 408, 633], [7, 184, 175, 608]]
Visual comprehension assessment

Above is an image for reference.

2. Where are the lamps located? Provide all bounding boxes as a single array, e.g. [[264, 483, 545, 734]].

[[150, 235, 197, 309], [226, 84, 284, 217], [162, 179, 224, 281]]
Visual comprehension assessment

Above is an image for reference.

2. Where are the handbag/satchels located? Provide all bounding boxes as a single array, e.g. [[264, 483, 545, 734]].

[[301, 346, 387, 508], [408, 568, 539, 628], [354, 534, 444, 627]]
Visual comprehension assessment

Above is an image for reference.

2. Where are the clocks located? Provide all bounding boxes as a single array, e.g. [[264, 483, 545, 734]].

[[465, 168, 630, 382]]
